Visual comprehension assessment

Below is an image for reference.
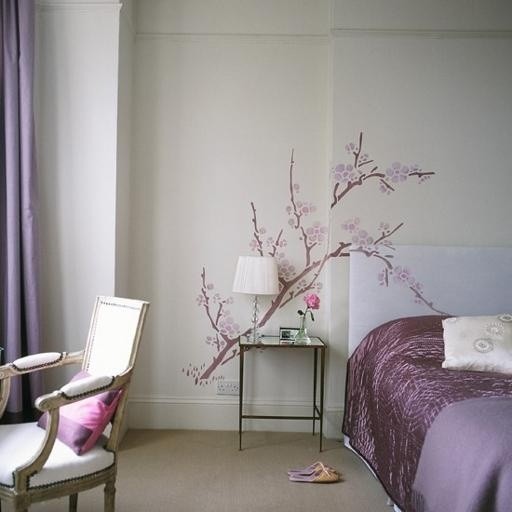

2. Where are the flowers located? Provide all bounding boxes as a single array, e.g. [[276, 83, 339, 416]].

[[297, 294, 320, 322]]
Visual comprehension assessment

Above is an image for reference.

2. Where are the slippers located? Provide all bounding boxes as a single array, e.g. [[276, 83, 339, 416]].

[[288, 462, 336, 476], [289, 468, 339, 482]]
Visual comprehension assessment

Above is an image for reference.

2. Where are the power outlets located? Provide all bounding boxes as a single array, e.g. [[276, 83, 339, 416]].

[[216, 382, 240, 396]]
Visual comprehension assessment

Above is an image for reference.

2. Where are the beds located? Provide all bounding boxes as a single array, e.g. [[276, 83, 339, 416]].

[[342, 245, 512, 512]]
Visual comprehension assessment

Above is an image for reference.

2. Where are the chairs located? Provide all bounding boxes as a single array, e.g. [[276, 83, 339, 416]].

[[1, 294, 150, 512]]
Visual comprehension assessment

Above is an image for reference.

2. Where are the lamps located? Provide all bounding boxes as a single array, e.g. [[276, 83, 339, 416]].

[[232, 255, 280, 343]]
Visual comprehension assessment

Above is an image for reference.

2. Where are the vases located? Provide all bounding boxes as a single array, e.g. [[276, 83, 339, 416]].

[[294, 316, 312, 345]]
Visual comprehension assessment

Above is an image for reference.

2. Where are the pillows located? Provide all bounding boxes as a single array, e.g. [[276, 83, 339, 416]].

[[36, 370, 124, 456], [441, 313, 512, 376]]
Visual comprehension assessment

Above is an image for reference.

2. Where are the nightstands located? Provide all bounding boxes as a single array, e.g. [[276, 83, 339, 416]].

[[239, 336, 328, 452]]
